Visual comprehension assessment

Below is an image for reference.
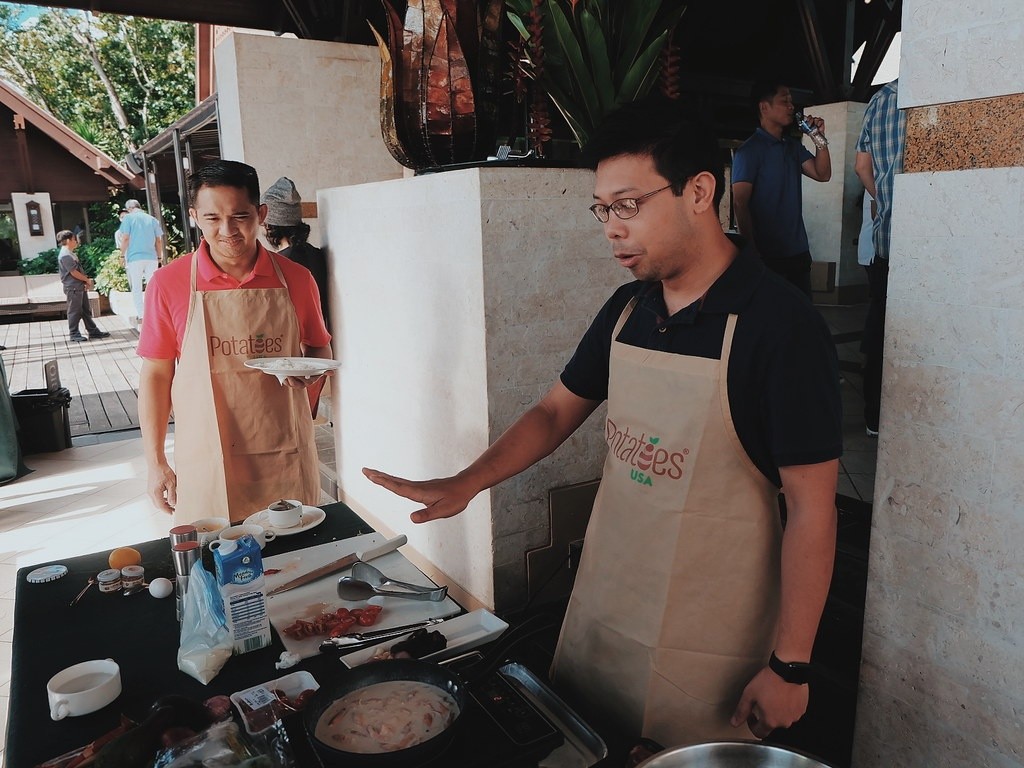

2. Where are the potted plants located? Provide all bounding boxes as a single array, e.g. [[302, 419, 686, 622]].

[[92, 251, 147, 316]]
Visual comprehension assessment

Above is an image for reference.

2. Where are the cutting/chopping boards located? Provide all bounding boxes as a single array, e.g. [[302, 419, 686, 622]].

[[260, 531, 461, 661]]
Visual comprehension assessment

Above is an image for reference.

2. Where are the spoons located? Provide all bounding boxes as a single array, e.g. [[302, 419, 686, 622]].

[[123, 577, 176, 596]]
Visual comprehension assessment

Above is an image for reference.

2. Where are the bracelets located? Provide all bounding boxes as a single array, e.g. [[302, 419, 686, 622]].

[[158, 258, 161, 259], [120, 256, 124, 258]]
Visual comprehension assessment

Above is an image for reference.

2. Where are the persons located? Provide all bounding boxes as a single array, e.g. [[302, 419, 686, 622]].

[[134, 160, 333, 528], [118, 200, 164, 331], [76, 223, 85, 244], [114, 210, 132, 290], [732, 85, 832, 302], [362, 105, 846, 750], [853, 79, 899, 435], [56, 231, 110, 341], [262, 177, 332, 419]]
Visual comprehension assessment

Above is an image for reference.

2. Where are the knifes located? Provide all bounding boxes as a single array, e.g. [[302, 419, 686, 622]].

[[265, 533, 409, 598]]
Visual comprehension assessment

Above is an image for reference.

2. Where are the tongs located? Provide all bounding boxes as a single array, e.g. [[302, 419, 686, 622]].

[[317, 619, 445, 655], [337, 560, 448, 602]]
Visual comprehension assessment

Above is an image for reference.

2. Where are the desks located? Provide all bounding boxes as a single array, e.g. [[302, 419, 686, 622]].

[[4, 502, 630, 768]]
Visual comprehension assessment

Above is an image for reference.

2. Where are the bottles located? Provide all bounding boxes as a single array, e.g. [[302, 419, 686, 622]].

[[796, 113, 828, 150], [168, 525, 201, 634]]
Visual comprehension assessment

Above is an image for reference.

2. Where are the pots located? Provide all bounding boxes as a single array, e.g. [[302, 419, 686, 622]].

[[301, 609, 563, 768]]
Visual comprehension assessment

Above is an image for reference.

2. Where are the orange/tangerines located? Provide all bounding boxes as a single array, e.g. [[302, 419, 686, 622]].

[[109, 547, 141, 571]]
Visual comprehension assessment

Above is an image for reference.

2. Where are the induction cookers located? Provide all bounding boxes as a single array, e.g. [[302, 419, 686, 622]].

[[281, 649, 563, 768]]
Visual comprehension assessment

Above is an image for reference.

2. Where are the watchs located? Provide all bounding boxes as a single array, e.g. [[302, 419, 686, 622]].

[[769, 649, 813, 685]]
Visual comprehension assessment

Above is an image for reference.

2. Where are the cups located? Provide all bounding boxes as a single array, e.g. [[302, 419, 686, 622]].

[[268, 499, 303, 528]]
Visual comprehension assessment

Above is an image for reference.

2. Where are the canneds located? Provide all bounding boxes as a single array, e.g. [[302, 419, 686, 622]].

[[97, 568, 122, 593], [122, 566, 144, 589]]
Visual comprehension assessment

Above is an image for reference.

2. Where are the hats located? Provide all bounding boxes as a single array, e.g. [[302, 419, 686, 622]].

[[260, 177, 302, 227], [118, 208, 129, 215]]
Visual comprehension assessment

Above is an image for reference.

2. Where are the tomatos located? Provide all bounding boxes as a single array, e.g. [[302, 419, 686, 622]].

[[238, 688, 315, 729], [283, 604, 382, 640]]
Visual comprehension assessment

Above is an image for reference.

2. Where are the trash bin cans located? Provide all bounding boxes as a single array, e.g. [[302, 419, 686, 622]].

[[10, 388, 72, 452], [0, 345, 21, 485]]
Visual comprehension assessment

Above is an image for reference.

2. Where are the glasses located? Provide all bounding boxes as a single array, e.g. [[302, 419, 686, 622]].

[[590, 176, 696, 223]]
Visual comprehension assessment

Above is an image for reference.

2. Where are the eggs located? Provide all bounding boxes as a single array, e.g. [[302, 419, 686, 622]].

[[149, 578, 173, 598]]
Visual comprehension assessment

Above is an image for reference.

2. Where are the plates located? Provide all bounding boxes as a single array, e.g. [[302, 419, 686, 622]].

[[243, 357, 340, 376], [242, 505, 326, 536]]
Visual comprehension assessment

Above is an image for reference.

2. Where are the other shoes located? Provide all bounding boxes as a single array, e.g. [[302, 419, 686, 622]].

[[866, 422, 878, 439], [89, 331, 109, 338], [70, 335, 87, 341]]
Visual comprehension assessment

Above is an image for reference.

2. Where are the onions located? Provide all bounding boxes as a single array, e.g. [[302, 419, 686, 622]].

[[162, 696, 233, 749]]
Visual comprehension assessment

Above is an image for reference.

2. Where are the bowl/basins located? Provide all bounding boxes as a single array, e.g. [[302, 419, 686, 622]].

[[229, 670, 320, 740], [47, 657, 122, 721], [635, 740, 835, 768], [191, 517, 230, 549], [208, 525, 276, 550]]
